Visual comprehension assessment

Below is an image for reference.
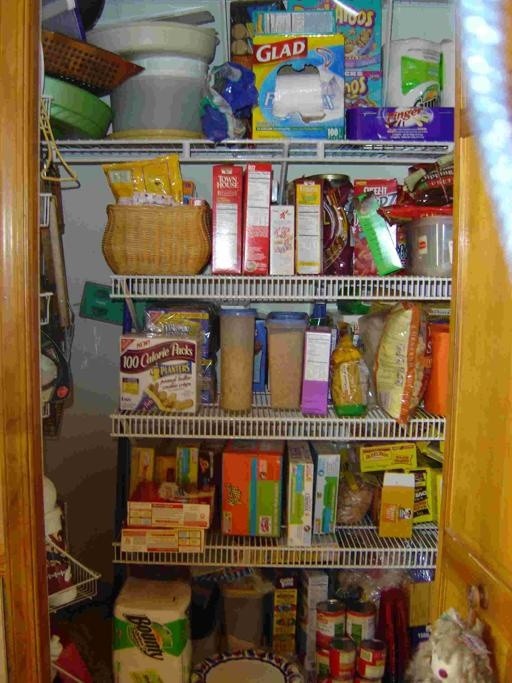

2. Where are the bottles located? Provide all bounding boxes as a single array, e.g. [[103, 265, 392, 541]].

[[220, 300, 308, 411], [221, 587, 264, 650], [373, 586, 411, 673]]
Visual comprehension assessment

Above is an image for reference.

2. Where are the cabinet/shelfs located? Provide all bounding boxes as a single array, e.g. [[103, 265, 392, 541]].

[[38, 43, 457, 683]]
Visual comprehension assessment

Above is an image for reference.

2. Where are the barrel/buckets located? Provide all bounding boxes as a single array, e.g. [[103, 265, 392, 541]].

[[111, 53, 209, 132]]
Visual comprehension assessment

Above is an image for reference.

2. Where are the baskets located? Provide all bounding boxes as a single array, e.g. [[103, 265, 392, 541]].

[[41, 32, 146, 99], [101, 205, 212, 277]]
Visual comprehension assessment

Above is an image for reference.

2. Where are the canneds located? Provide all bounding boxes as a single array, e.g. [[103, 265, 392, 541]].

[[316, 599, 388, 683]]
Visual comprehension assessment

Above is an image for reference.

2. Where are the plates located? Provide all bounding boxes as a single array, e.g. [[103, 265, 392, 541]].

[[87, 22, 217, 62]]
